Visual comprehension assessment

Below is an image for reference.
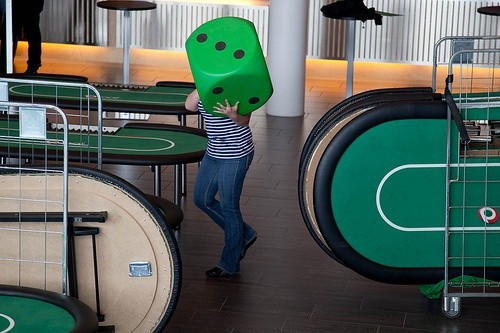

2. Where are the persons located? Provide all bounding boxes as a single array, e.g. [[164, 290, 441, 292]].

[[184, 88, 259, 278], [0, 0, 44, 73]]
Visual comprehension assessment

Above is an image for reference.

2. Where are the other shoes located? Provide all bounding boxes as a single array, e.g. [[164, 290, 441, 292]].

[[205, 266, 240, 280], [24, 68, 37, 75], [238, 234, 257, 261]]
